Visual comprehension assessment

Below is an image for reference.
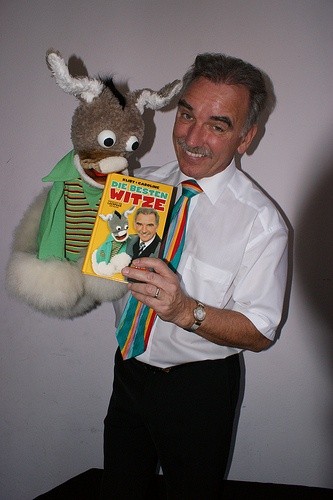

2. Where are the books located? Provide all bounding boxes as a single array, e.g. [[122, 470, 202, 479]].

[[81, 171, 174, 285]]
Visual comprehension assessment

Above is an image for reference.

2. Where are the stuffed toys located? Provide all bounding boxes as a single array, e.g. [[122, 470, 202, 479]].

[[6, 49, 183, 319]]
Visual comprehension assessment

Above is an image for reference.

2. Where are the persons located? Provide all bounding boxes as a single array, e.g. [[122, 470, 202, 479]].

[[100, 51, 289, 500]]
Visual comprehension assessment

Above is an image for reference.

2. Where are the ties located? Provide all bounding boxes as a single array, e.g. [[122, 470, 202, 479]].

[[116, 181, 203, 361]]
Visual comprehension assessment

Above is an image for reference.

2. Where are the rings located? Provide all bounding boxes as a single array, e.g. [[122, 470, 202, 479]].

[[154, 287, 160, 297]]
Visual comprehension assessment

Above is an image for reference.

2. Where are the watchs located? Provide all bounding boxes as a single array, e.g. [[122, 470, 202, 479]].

[[183, 298, 207, 333]]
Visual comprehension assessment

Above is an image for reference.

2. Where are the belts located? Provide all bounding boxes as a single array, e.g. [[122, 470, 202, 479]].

[[134, 357, 229, 375]]
